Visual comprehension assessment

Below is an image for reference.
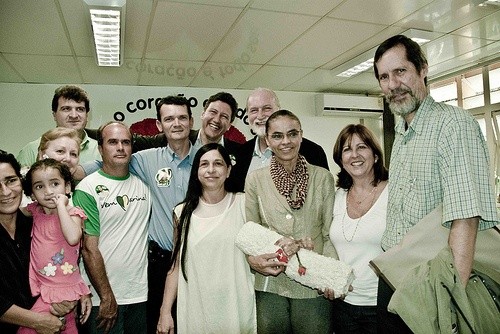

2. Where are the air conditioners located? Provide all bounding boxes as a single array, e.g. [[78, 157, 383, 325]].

[[314, 93, 385, 119]]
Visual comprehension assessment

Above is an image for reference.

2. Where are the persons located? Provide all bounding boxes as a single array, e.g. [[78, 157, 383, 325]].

[[72, 121, 153, 334], [69, 96, 200, 334], [243, 109, 340, 334], [16, 158, 88, 334], [131, 92, 239, 157], [0, 149, 93, 334], [36, 127, 82, 173], [15, 85, 104, 185], [328, 124, 389, 334], [155, 142, 258, 334], [373, 34, 500, 334], [224, 87, 329, 194]]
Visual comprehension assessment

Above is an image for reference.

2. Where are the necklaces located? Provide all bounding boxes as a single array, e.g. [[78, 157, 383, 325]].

[[351, 187, 377, 205], [342, 185, 379, 242]]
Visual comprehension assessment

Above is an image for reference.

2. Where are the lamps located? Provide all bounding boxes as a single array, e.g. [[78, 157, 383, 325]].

[[329, 26, 436, 81], [82, 0, 129, 67]]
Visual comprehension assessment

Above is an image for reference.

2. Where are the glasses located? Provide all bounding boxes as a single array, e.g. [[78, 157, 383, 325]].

[[0, 176, 24, 189], [266, 131, 301, 140]]
